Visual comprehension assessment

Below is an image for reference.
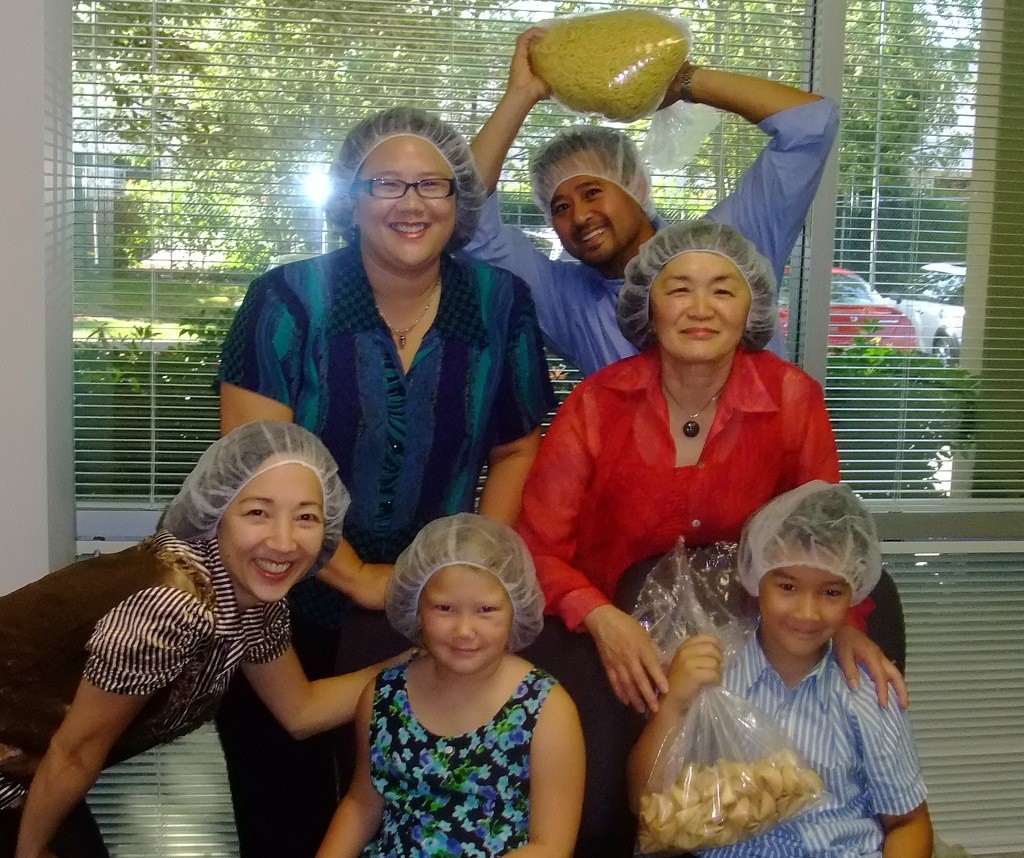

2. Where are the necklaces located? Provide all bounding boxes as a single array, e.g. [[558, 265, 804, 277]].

[[661, 374, 723, 436], [377, 270, 442, 348]]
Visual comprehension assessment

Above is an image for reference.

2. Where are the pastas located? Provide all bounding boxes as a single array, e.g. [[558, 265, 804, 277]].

[[527, 9, 689, 122]]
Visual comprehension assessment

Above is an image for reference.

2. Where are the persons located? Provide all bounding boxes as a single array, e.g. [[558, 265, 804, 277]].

[[627, 480, 937, 858], [215, 26, 914, 712], [0, 422, 431, 858], [316, 514, 585, 858]]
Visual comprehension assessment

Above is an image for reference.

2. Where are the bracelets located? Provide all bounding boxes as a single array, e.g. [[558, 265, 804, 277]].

[[411, 645, 422, 659], [680, 65, 698, 104]]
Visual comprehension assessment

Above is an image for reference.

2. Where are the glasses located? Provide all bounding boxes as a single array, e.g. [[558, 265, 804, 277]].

[[359, 178, 456, 199]]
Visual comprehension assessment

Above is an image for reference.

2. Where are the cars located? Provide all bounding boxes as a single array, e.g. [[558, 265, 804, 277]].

[[521, 226, 581, 266], [882, 263, 967, 367], [777, 265, 917, 354]]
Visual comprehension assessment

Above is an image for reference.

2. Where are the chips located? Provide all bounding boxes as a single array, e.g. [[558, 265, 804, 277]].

[[639, 751, 823, 851]]
